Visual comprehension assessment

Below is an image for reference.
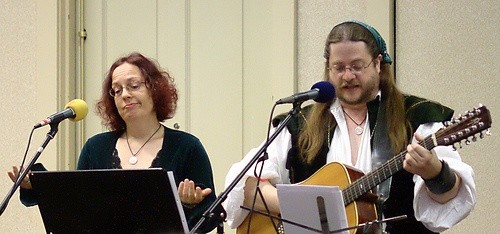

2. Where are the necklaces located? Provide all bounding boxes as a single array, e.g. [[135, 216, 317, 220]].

[[342, 107, 367, 136], [123, 123, 162, 165]]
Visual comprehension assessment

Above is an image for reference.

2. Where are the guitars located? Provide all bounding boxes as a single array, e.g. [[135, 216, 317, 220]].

[[234, 103, 493, 234]]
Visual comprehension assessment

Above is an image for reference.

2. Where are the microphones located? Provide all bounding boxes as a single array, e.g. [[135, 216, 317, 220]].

[[34, 99, 89, 128], [276, 81, 336, 105]]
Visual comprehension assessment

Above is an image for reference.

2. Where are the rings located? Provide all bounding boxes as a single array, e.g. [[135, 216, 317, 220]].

[[24, 178, 30, 184]]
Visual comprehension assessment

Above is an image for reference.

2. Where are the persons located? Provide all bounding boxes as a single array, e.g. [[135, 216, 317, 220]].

[[6, 51, 228, 234], [223, 20, 477, 234]]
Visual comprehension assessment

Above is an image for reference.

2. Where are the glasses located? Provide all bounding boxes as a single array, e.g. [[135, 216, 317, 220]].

[[109, 80, 151, 97], [327, 58, 374, 74]]
